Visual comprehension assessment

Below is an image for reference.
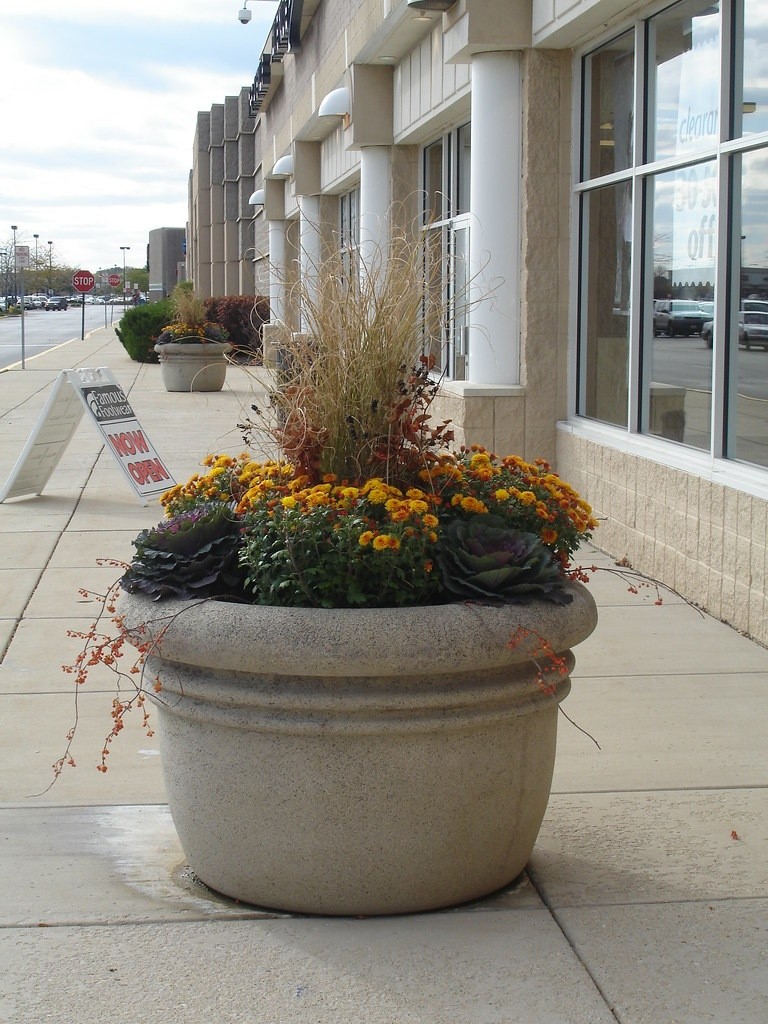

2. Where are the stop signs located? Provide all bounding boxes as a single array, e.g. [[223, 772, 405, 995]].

[[108, 274, 121, 288], [71, 270, 95, 294]]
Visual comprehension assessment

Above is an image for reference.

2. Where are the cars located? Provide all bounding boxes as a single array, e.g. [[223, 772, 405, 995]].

[[0, 289, 149, 313], [653, 299, 713, 337], [701, 299, 768, 352]]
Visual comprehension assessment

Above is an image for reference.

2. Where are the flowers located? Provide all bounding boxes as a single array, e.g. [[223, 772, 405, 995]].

[[21, 188, 707, 798], [155, 280, 234, 346]]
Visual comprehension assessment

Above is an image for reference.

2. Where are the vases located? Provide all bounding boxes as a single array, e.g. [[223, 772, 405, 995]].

[[111, 573, 597, 917], [154, 342, 234, 392]]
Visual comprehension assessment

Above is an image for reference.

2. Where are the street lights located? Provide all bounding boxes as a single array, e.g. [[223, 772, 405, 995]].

[[120, 247, 130, 301]]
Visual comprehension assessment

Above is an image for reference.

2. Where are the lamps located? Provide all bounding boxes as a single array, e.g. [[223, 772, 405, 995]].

[[407, 0, 456, 13], [318, 87, 350, 117], [248, 189, 265, 205], [272, 156, 293, 175]]
